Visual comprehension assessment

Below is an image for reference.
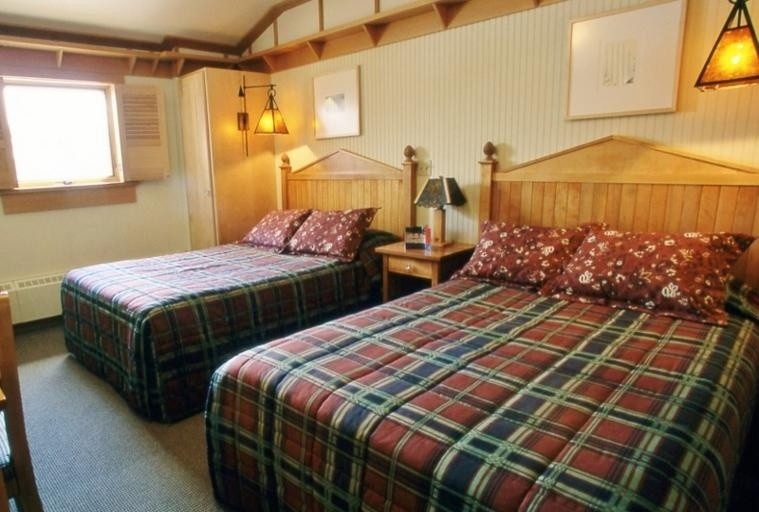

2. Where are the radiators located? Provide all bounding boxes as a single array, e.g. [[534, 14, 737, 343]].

[[1, 274, 64, 326]]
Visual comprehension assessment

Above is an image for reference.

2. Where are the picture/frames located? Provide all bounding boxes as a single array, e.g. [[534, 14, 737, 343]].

[[313, 65, 361, 139], [563, 0, 690, 121]]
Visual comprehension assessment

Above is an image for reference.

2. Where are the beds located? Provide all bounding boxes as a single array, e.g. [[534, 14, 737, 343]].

[[62, 136, 419, 427], [201, 136, 757, 511]]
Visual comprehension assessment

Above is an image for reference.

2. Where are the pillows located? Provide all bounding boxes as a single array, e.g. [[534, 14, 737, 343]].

[[278, 207, 382, 263], [235, 209, 312, 255], [541, 229, 758, 326], [449, 216, 610, 292]]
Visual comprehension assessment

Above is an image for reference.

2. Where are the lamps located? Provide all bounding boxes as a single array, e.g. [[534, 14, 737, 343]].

[[237, 74, 290, 158], [413, 174, 468, 247], [693, 0, 759, 96]]
